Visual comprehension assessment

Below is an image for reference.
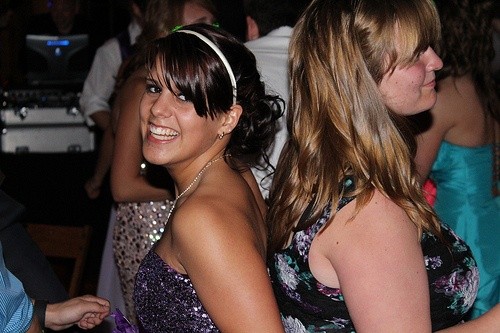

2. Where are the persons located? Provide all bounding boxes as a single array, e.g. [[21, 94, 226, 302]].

[[0, 239, 111, 333], [80, 0, 500, 333], [39, 0, 89, 38]]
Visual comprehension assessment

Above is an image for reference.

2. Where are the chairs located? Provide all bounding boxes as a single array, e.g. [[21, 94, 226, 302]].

[[23, 223, 91, 300]]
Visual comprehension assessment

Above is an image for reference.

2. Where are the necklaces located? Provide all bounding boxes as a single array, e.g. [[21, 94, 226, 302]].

[[165, 153, 235, 226]]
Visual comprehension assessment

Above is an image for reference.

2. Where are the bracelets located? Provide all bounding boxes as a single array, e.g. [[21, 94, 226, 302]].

[[34, 297, 49, 331]]
